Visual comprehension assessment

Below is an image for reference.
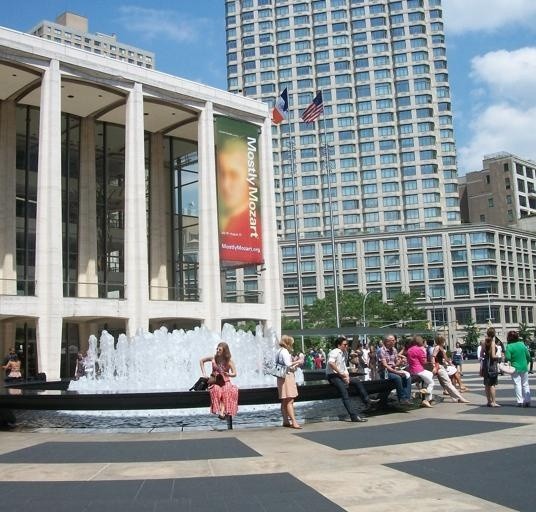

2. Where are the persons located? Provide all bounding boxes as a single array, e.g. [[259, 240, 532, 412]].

[[291, 335, 470, 422], [4, 348, 17, 377], [477, 328, 533, 407], [0, 354, 22, 395], [275, 335, 304, 429], [200, 342, 238, 419], [74, 351, 85, 379]]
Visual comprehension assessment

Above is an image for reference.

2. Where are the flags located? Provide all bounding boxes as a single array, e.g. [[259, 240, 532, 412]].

[[302, 91, 324, 124], [276, 88, 288, 111], [272, 107, 283, 125]]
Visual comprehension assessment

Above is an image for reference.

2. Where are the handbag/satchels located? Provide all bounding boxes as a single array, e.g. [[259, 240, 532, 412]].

[[265, 360, 287, 379], [499, 363, 516, 373], [189, 377, 208, 391]]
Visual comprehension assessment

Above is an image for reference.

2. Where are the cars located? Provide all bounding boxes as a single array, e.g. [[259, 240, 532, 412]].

[[464, 341, 536, 360]]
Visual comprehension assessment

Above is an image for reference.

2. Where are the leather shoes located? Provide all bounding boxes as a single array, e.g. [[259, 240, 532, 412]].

[[487, 402, 501, 407], [422, 400, 433, 408], [367, 398, 379, 405], [400, 400, 415, 405], [458, 398, 469, 403], [352, 416, 368, 422]]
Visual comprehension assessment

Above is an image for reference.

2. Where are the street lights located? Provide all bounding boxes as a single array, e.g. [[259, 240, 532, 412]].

[[360, 288, 382, 345], [434, 319, 461, 332], [418, 292, 436, 335]]
